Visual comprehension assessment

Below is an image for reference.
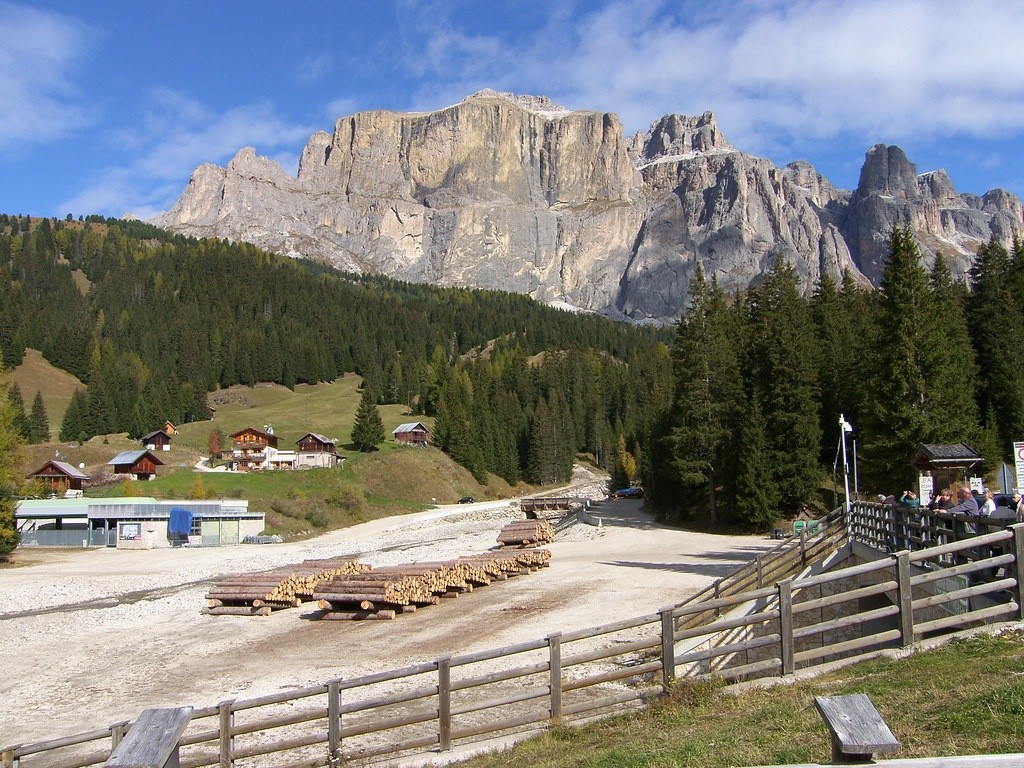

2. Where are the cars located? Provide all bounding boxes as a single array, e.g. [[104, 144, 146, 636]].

[[614, 487, 641, 499], [457, 496, 475, 504]]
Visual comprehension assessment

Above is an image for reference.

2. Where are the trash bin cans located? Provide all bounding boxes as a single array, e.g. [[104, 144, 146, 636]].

[[792, 521, 806, 538], [808, 520, 822, 536]]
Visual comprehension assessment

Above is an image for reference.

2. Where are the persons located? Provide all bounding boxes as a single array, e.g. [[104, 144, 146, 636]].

[[1011, 492, 1024, 523], [877, 494, 898, 506], [933, 488, 954, 509], [971, 490, 981, 511], [979, 488, 996, 516], [934, 487, 979, 553], [988, 496, 1016, 575], [899, 491, 921, 550]]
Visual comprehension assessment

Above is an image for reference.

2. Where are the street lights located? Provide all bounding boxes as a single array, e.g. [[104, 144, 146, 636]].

[[838, 414, 853, 543]]
